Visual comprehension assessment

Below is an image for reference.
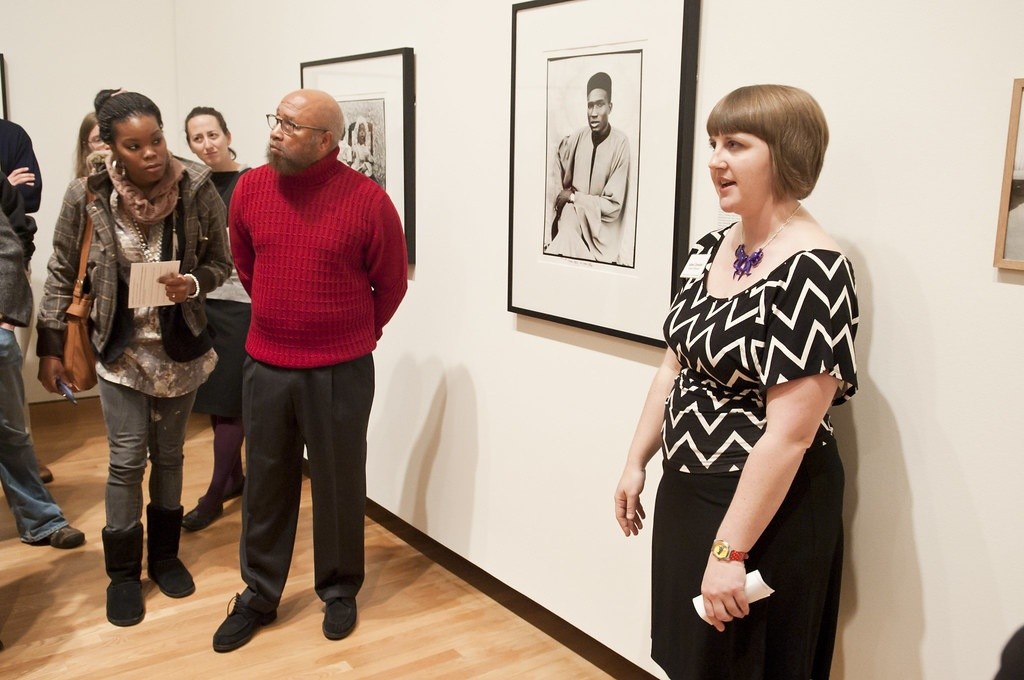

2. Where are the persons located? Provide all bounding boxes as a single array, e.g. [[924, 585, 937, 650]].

[[544, 73, 632, 263], [182, 102, 255, 530], [353, 123, 375, 177], [0, 117, 84, 549], [615, 84, 859, 680], [212, 87, 409, 653], [75, 112, 114, 178], [35, 90, 233, 627]]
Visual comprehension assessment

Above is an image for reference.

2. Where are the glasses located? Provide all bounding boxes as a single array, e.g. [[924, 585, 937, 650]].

[[266, 113, 330, 136]]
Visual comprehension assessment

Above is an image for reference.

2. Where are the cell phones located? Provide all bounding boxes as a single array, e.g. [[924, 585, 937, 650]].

[[57, 381, 78, 404]]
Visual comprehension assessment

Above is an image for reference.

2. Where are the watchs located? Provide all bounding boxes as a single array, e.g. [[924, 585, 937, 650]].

[[710, 539, 748, 562]]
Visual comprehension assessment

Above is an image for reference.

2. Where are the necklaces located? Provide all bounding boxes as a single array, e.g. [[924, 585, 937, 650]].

[[119, 204, 163, 263], [732, 199, 802, 281]]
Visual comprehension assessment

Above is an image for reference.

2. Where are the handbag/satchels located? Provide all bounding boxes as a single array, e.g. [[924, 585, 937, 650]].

[[63, 291, 99, 394]]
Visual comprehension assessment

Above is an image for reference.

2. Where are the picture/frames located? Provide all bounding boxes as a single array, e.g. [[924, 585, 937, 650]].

[[993, 79, 1024, 271], [507, 0, 700, 351], [300, 46, 415, 265]]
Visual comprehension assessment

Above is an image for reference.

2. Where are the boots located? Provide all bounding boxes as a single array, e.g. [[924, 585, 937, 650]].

[[102, 524, 146, 628], [146, 502, 195, 599]]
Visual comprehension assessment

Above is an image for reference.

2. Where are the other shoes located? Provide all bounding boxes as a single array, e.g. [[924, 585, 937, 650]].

[[39, 465, 54, 483], [183, 504, 224, 530], [221, 475, 245, 502]]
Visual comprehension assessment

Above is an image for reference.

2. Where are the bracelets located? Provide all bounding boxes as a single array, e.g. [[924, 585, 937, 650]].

[[184, 274, 200, 298]]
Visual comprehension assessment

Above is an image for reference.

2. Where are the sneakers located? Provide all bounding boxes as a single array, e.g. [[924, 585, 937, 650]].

[[213, 593, 278, 653], [46, 525, 85, 549], [322, 598, 357, 640]]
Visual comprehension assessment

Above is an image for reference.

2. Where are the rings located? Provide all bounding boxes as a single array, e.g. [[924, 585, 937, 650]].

[[172, 292, 175, 298]]
